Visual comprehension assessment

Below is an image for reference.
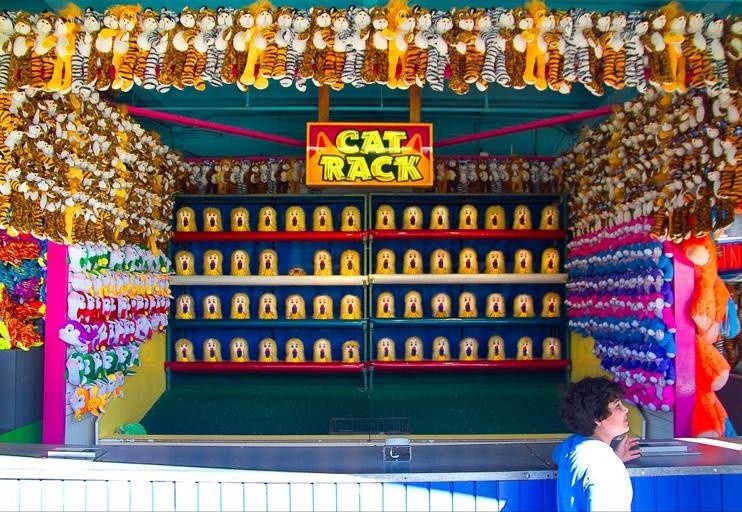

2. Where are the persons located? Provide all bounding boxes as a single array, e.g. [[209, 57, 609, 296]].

[[553, 377, 641, 512]]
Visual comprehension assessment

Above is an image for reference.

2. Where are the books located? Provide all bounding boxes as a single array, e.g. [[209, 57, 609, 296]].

[[643, 448, 701, 456], [639, 441, 688, 453], [47, 447, 104, 458]]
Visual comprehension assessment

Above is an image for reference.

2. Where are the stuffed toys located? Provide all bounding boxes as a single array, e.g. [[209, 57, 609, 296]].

[[175, 153, 565, 195], [0, 86, 182, 419], [0, 0, 742, 99], [0, 0, 740, 439], [552, 85, 740, 439]]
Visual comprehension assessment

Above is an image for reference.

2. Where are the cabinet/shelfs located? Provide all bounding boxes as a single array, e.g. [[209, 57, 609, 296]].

[[367, 192, 570, 394], [1, 348, 45, 431], [164, 192, 368, 389]]
